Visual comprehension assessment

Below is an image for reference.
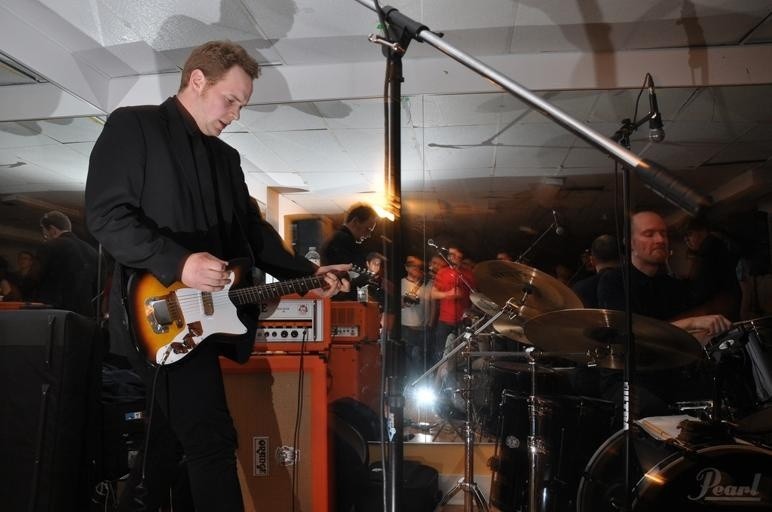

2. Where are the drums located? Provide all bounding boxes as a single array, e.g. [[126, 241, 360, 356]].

[[706, 315, 768, 418], [578, 413, 767, 512], [490, 389, 619, 511]]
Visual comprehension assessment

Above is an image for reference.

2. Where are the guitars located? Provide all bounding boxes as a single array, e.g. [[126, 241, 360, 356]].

[[124, 255, 380, 366]]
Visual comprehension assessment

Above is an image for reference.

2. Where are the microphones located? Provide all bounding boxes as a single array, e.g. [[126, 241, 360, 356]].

[[553, 208, 564, 234], [427, 238, 446, 252], [648, 76, 666, 144]]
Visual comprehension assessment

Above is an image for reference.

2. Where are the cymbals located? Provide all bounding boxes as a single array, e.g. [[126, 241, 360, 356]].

[[473, 261, 583, 319], [524, 308, 705, 369], [492, 307, 548, 348]]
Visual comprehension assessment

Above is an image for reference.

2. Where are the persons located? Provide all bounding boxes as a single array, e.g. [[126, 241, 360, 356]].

[[85, 39, 353, 512], [304, 206, 772, 430], [0, 209, 108, 319]]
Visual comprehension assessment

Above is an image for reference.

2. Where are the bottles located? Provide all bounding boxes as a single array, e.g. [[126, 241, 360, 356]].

[[356, 286, 369, 303], [304, 246, 320, 267]]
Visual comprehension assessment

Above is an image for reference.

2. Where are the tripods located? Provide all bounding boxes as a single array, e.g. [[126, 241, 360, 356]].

[[439, 376, 485, 510]]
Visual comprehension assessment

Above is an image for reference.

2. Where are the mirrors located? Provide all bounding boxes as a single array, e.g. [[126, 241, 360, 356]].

[[0, 74, 771, 449]]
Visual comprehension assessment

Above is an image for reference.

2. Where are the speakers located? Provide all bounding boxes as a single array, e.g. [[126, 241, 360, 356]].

[[1, 311, 104, 511]]
